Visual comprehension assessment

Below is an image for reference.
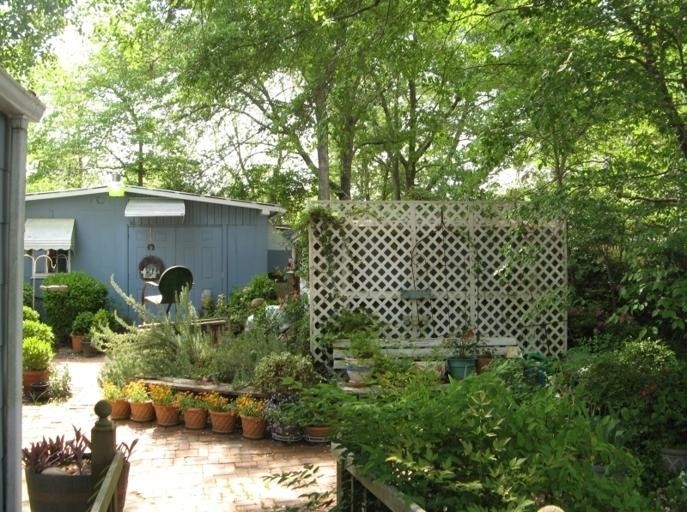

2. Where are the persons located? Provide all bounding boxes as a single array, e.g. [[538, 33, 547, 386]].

[[245, 297, 292, 333]]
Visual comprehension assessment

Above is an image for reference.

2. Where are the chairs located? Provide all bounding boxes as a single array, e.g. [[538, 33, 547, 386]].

[[141, 265, 193, 324]]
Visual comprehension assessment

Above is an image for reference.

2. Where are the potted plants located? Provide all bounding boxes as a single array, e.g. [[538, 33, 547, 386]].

[[68, 308, 112, 359], [22, 336, 58, 395], [29, 380, 51, 404], [99, 351, 357, 449], [20, 423, 139, 512], [341, 332, 506, 391]]
